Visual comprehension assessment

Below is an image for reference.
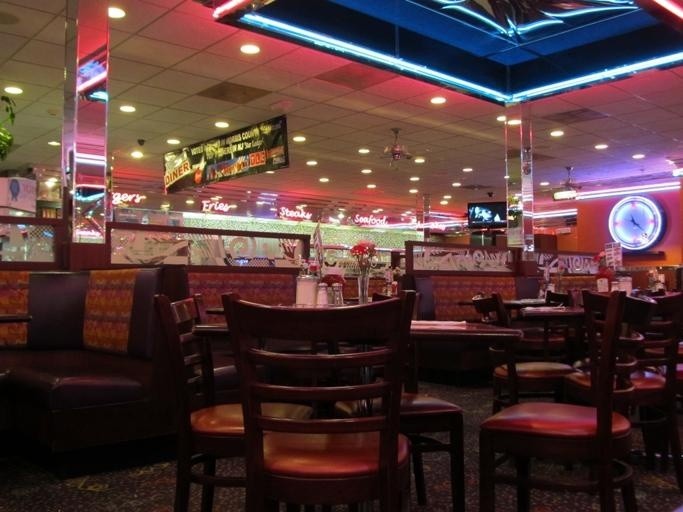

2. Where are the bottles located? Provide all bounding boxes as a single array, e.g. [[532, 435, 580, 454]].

[[316, 283, 330, 306], [332, 284, 345, 305], [611, 282, 620, 295]]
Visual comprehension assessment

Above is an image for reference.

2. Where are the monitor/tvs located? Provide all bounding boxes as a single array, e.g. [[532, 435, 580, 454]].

[[467, 202, 508, 229]]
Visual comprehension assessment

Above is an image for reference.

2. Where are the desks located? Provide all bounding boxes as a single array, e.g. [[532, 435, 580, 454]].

[[521, 304, 584, 360], [190, 320, 524, 415]]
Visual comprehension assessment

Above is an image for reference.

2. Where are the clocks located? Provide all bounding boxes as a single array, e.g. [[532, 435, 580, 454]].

[[606, 194, 664, 251]]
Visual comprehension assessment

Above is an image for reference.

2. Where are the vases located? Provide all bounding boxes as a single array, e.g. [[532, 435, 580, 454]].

[[356, 270, 371, 305]]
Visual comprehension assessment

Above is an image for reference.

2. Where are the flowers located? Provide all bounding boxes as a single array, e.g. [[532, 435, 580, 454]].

[[351, 238, 378, 273]]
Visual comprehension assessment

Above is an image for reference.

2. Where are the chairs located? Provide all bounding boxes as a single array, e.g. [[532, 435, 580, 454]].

[[317, 290, 468, 512], [477, 289, 636, 512], [224, 291, 418, 512], [565, 290, 658, 409], [633, 295, 683, 495], [472, 292, 580, 411], [152, 293, 247, 512]]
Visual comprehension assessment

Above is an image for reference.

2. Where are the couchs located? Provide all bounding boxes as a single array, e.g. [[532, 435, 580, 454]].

[[0, 266, 164, 462], [158, 264, 273, 395]]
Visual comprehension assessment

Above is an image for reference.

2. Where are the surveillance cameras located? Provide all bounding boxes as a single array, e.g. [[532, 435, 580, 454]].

[[137, 139, 145, 146], [488, 192, 494, 198]]
